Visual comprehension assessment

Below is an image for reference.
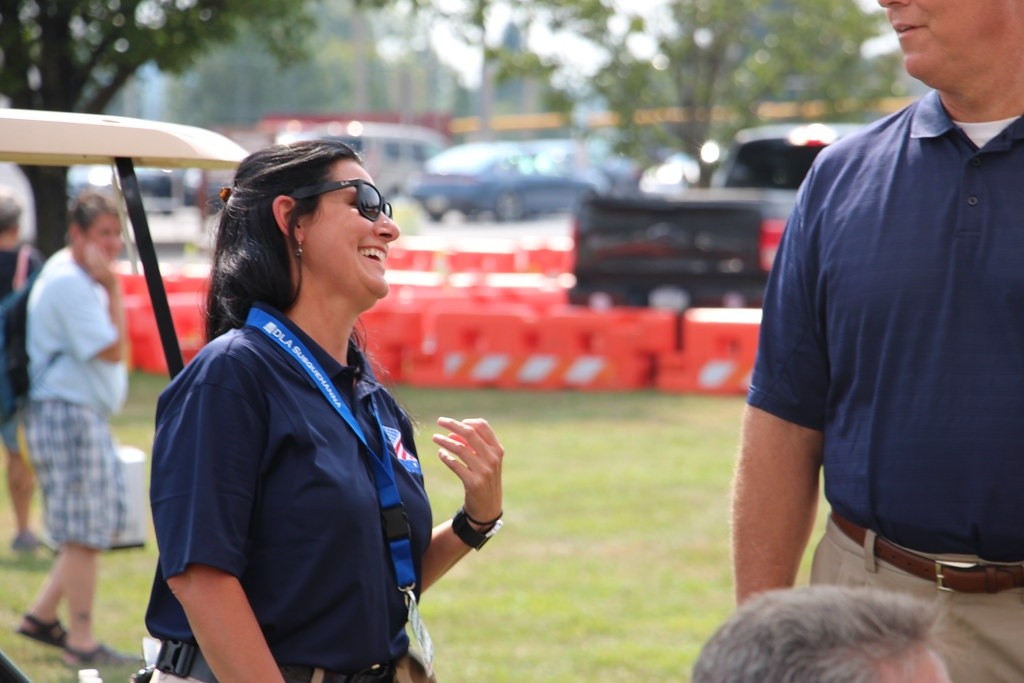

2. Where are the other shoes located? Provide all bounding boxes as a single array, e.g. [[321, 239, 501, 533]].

[[14, 534, 47, 552]]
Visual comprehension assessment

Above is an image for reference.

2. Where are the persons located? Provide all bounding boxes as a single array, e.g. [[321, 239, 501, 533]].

[[17, 195, 130, 668], [694, 584, 1024, 683], [0, 197, 47, 550], [733, 1, 1024, 683], [131, 141, 503, 682]]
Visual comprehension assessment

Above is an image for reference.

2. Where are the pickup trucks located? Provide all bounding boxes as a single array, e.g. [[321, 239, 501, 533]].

[[568, 124, 869, 311]]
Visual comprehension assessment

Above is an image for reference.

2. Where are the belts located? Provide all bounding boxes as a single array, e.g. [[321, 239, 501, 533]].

[[155, 638, 393, 683], [831, 511, 1024, 593]]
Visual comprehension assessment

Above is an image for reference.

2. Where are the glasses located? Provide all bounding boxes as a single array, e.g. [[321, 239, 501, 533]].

[[289, 179, 393, 221]]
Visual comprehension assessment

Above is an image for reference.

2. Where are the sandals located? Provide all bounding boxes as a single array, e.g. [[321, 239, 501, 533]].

[[63, 646, 145, 670], [17, 615, 67, 647]]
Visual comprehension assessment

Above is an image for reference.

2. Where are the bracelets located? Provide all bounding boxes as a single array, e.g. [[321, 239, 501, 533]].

[[462, 510, 503, 526]]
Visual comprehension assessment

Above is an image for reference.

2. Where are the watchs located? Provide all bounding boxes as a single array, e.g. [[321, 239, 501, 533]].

[[452, 511, 502, 552]]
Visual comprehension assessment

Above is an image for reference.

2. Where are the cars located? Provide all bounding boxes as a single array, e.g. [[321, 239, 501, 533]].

[[410, 139, 609, 222]]
